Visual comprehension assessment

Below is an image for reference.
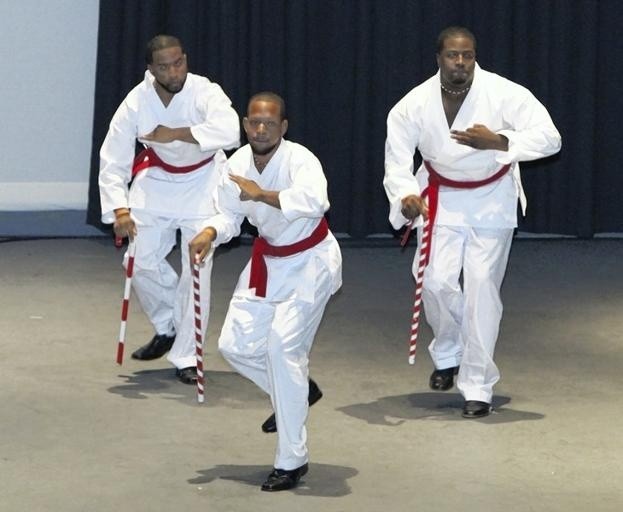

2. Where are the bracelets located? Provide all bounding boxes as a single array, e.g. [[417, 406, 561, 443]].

[[115, 212, 130, 219]]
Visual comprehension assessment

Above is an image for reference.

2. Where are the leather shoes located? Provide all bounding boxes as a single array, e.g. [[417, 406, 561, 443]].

[[430, 367, 460, 390], [262, 464, 308, 491], [130, 333, 175, 360], [461, 401, 491, 418], [176, 368, 198, 383], [262, 379, 322, 433]]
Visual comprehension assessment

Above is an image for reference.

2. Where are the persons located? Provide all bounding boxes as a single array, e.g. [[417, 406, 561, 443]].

[[185, 90, 350, 493], [95, 36, 241, 386], [382, 26, 565, 420]]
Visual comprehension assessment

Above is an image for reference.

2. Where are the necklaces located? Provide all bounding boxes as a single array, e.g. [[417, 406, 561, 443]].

[[253, 152, 269, 167], [440, 82, 471, 97]]
